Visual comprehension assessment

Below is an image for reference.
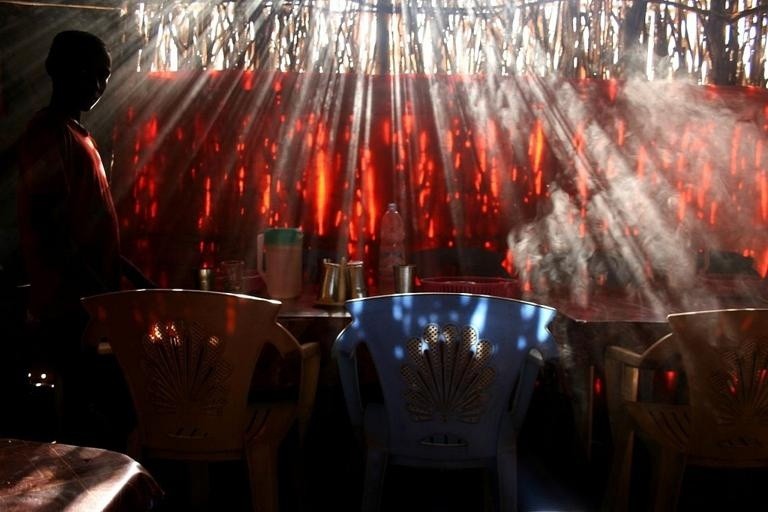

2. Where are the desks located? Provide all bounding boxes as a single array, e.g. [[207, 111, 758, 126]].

[[0, 438, 167, 512], [264, 274, 763, 464]]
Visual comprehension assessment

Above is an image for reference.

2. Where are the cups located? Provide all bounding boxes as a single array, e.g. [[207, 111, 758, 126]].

[[257, 228, 305, 300], [195, 266, 213, 291], [221, 260, 245, 292]]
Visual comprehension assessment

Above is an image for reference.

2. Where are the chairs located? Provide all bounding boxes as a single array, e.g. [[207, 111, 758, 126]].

[[600, 307, 768, 511], [332, 290, 574, 511], [79, 286, 322, 512]]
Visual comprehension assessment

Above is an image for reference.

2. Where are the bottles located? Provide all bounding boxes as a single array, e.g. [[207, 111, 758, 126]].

[[320, 261, 346, 302], [393, 263, 416, 294], [379, 203, 407, 277], [348, 261, 368, 299]]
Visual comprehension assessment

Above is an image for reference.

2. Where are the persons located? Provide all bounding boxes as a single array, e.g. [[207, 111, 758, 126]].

[[21, 28, 157, 412]]
